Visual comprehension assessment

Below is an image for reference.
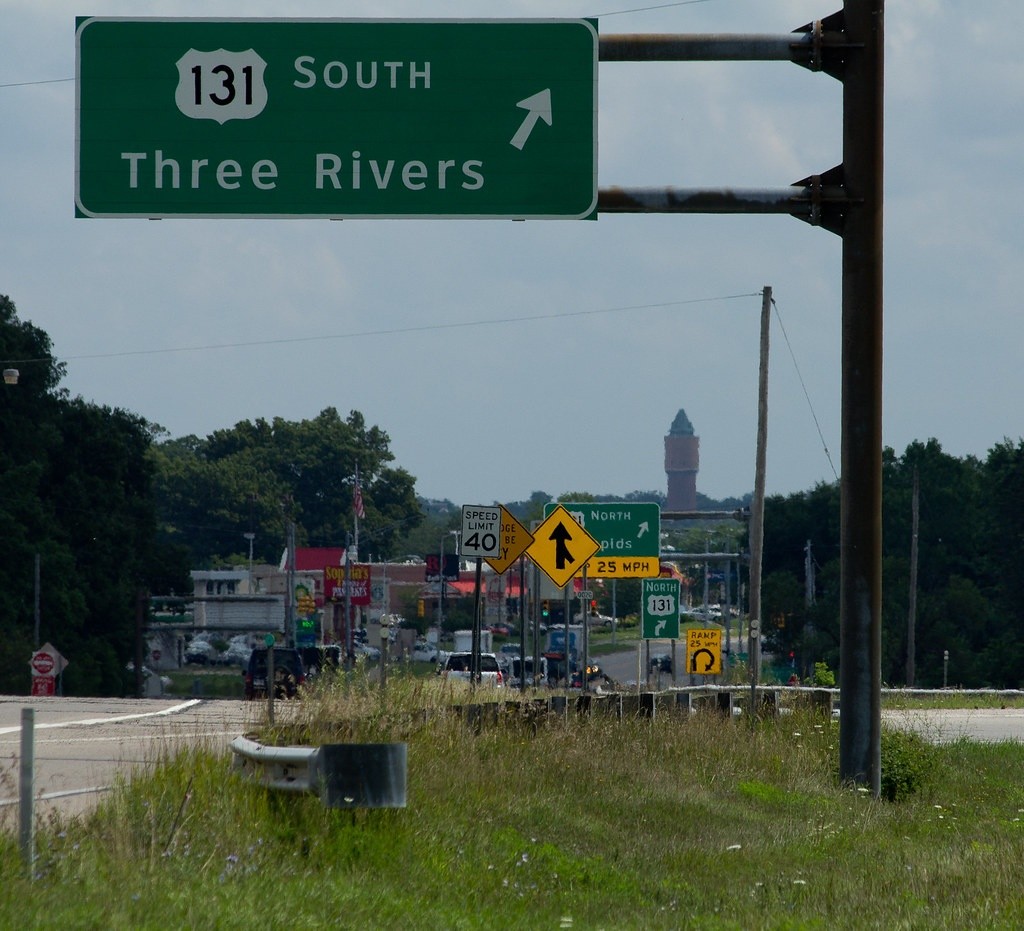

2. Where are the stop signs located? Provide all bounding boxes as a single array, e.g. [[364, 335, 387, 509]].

[[152, 650, 161, 661], [31, 651, 55, 676]]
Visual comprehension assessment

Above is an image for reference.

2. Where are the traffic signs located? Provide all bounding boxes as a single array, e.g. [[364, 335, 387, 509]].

[[74, 16, 600, 219], [686, 629, 721, 673], [543, 502, 660, 579], [640, 577, 680, 640]]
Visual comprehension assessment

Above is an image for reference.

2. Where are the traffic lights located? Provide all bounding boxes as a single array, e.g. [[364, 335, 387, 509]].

[[542, 601, 549, 618], [590, 600, 597, 606]]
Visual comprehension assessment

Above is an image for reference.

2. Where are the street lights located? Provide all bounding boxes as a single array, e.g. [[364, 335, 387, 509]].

[[244, 532, 255, 595], [379, 555, 424, 695], [346, 531, 358, 655]]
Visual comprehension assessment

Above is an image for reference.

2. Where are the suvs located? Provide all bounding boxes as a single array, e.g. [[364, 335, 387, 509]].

[[243, 648, 305, 700], [441, 654, 504, 688]]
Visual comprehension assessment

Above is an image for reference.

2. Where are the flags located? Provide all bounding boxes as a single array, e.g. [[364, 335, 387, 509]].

[[355, 485, 365, 519]]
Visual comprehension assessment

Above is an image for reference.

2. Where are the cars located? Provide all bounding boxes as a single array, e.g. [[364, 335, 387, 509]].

[[184, 619, 446, 677], [573, 610, 618, 627], [647, 653, 671, 673], [682, 605, 739, 623], [431, 609, 619, 688]]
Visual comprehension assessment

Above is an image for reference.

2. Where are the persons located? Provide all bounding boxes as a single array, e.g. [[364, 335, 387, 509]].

[[123, 658, 136, 699], [353, 622, 367, 649]]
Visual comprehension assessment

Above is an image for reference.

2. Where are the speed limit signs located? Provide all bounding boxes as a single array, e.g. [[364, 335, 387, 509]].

[[461, 505, 502, 558]]
